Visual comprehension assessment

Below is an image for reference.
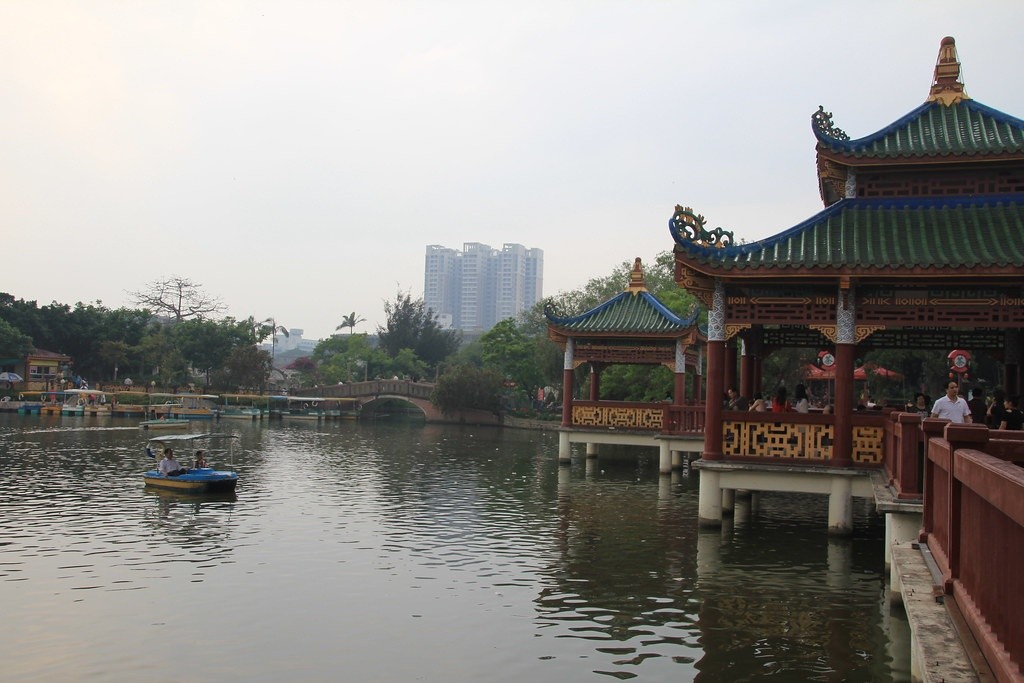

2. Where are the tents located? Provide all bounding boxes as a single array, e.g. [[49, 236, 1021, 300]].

[[854, 362, 906, 401], [794, 361, 837, 406]]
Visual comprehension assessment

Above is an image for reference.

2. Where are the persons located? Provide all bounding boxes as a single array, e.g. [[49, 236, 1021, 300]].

[[193, 451, 209, 468], [723, 380, 1023, 431], [159, 448, 189, 477], [39, 376, 106, 405]]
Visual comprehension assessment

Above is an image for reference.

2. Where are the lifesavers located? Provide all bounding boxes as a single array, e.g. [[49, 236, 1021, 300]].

[[303, 403, 308, 409], [357, 404, 362, 410], [146, 440, 166, 458], [77, 398, 86, 405], [40, 394, 46, 401], [19, 394, 25, 401], [312, 401, 319, 406]]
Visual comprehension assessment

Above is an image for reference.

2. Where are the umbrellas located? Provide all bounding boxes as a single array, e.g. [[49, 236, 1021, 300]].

[[0, 372, 24, 389]]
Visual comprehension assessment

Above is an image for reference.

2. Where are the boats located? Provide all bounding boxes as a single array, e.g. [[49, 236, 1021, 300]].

[[142, 432, 239, 494], [142, 418, 193, 430]]
